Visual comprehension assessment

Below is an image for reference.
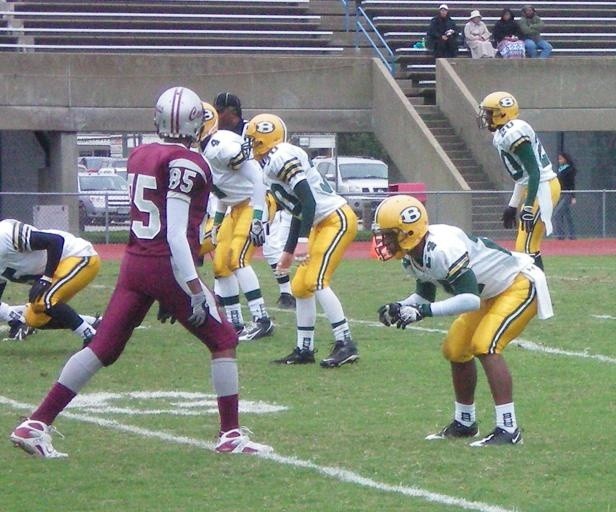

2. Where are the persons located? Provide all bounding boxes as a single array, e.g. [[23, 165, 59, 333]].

[[464, 9, 498, 59], [371, 195, 555, 446], [492, 8, 526, 59], [241, 114, 360, 368], [205, 92, 299, 310], [0, 216, 103, 351], [555, 151, 580, 241], [518, 5, 553, 59], [0, 279, 37, 343], [8, 86, 279, 458], [190, 101, 279, 343], [425, 5, 460, 58], [474, 91, 560, 272]]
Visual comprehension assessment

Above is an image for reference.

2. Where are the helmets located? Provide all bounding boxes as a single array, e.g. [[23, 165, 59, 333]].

[[482, 92, 519, 129], [373, 194, 428, 263], [155, 87, 286, 157]]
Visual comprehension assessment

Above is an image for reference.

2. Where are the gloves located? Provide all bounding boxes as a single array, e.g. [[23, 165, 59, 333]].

[[187, 291, 210, 327], [210, 225, 220, 245], [519, 211, 535, 234], [501, 206, 515, 229], [250, 219, 265, 247], [377, 302, 425, 328], [28, 275, 52, 303]]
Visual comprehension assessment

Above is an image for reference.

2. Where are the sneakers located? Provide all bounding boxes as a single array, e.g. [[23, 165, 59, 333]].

[[467, 425, 524, 448], [9, 417, 69, 460], [215, 428, 274, 455], [273, 294, 299, 310], [279, 341, 358, 365], [425, 420, 481, 439], [232, 323, 245, 337], [235, 316, 276, 340]]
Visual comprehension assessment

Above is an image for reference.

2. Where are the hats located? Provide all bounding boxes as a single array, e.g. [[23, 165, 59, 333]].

[[468, 10, 481, 20], [521, 5, 536, 12], [439, 4, 449, 11]]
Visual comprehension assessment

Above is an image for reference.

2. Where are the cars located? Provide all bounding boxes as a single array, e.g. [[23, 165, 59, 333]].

[[77, 149, 128, 174], [77, 176, 131, 226]]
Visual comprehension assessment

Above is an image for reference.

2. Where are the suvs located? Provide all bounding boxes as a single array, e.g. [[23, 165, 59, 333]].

[[309, 155, 389, 218]]
